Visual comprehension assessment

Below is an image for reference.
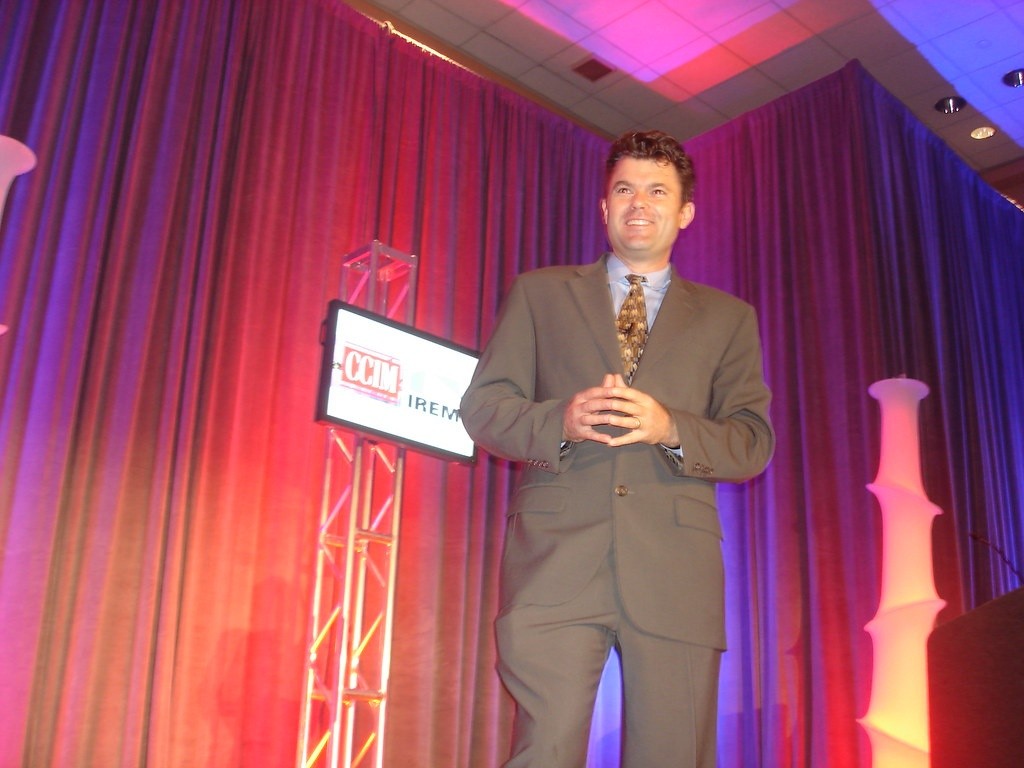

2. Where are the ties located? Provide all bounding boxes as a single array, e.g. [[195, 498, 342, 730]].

[[616, 274, 649, 386]]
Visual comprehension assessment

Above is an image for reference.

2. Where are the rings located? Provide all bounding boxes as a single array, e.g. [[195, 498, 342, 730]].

[[633, 417, 641, 430]]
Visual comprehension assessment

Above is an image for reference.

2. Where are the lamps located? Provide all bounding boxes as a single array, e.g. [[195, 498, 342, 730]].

[[856, 374, 946, 768]]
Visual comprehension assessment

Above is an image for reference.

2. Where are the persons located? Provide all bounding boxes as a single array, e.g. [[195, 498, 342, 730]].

[[459, 133, 775, 768]]
